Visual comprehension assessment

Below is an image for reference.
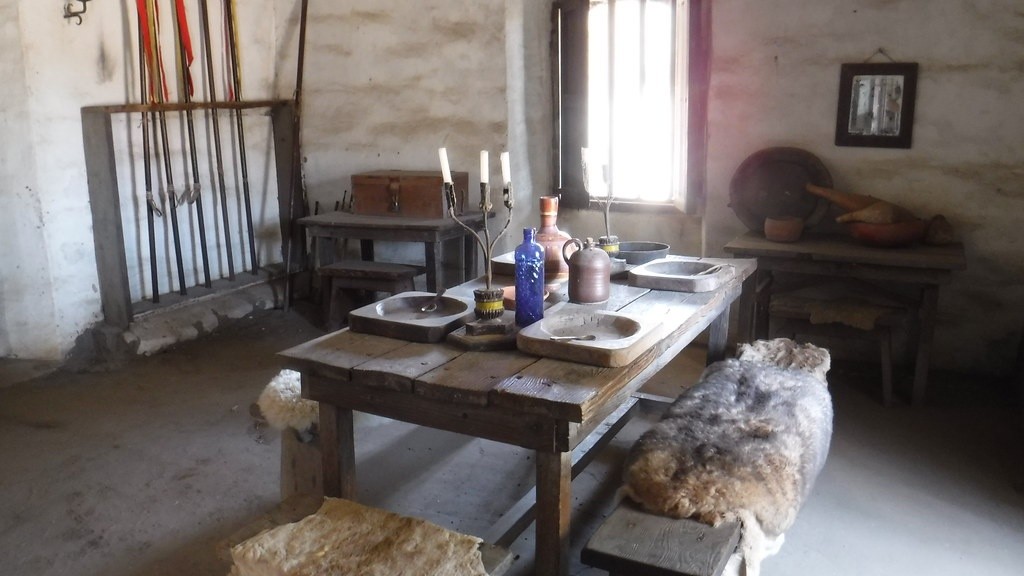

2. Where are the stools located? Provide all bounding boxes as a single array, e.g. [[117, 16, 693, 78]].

[[259, 367, 325, 496], [318, 260, 426, 335], [220, 496, 516, 576]]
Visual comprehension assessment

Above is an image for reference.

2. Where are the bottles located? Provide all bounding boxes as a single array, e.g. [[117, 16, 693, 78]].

[[533, 196, 575, 304], [515, 228, 544, 325], [563, 237, 609, 304]]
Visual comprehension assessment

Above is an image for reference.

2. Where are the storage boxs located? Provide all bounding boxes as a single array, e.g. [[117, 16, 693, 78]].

[[351, 169, 469, 219]]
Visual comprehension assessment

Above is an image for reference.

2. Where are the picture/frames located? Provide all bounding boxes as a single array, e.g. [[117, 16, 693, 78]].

[[834, 63, 919, 148]]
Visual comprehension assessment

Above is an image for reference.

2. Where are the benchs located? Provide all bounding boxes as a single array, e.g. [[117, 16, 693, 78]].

[[579, 338, 835, 576], [768, 286, 912, 408]]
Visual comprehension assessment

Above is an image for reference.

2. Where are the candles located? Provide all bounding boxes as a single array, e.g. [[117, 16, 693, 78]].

[[438, 148, 452, 183], [500, 152, 512, 186], [480, 149, 489, 183]]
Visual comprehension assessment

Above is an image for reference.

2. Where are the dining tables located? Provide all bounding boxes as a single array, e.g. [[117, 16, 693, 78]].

[[273, 248, 758, 576]]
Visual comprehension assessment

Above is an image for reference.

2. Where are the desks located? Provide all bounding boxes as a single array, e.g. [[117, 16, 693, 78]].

[[724, 229, 967, 412], [297, 210, 497, 329]]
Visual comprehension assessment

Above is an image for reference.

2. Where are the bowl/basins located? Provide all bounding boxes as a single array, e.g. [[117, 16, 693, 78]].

[[619, 240, 671, 265], [502, 286, 548, 309], [763, 218, 802, 242]]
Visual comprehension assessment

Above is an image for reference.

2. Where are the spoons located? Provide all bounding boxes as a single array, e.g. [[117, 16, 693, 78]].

[[421, 287, 447, 312], [552, 333, 596, 344]]
